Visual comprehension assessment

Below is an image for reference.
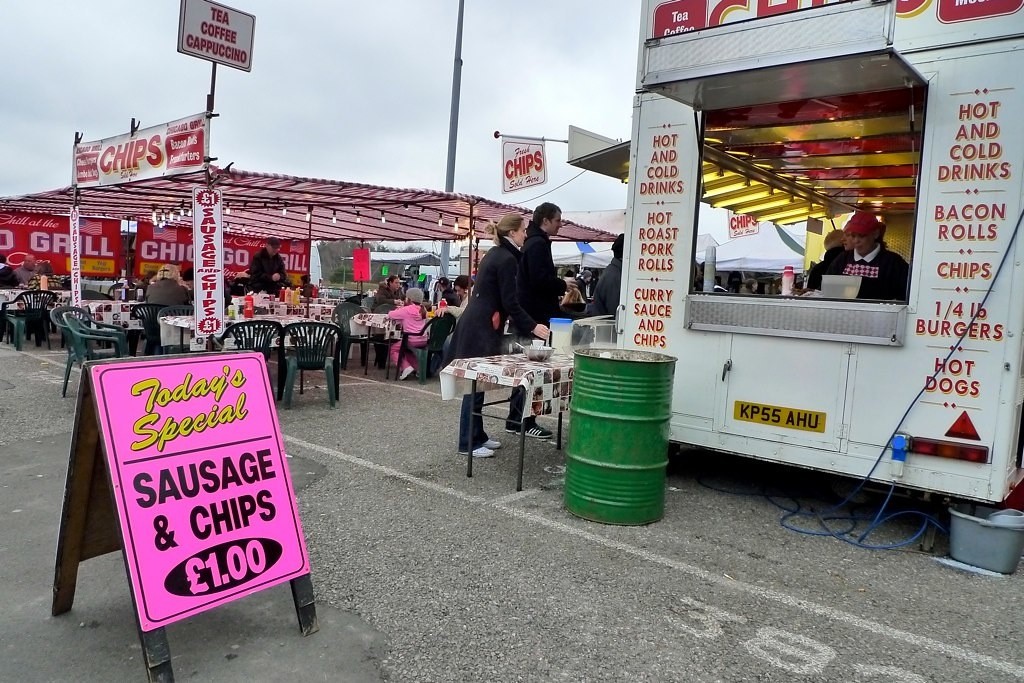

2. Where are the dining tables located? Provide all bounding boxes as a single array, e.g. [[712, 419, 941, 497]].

[[438, 350, 574, 492], [158, 315, 338, 356], [349, 312, 442, 378], [80, 298, 156, 359]]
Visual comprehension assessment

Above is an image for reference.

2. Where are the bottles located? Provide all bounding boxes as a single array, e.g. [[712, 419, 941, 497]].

[[227, 282, 319, 321], [137, 286, 144, 302], [782, 266, 794, 296], [439, 298, 447, 308], [367, 288, 377, 297], [431, 305, 436, 314], [121, 279, 129, 303], [40, 273, 48, 290], [339, 288, 345, 300], [703, 246, 716, 292]]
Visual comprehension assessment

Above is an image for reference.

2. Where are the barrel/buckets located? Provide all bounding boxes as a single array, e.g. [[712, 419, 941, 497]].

[[565, 348, 679, 527], [573, 320, 617, 345]]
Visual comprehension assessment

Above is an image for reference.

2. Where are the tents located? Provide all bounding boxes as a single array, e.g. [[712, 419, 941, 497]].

[[539, 205, 809, 277], [1, 155, 615, 330], [342, 246, 457, 291]]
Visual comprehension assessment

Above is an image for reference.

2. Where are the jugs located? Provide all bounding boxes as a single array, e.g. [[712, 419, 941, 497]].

[[546, 318, 573, 355]]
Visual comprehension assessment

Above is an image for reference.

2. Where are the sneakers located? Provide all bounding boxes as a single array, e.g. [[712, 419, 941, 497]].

[[481, 438, 502, 448], [505, 419, 553, 438], [398, 366, 415, 380], [460, 446, 496, 458]]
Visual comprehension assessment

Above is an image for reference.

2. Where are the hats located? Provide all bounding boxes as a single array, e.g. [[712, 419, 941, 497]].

[[437, 277, 449, 286], [842, 211, 877, 237], [405, 288, 423, 303], [157, 264, 180, 282], [266, 237, 281, 249]]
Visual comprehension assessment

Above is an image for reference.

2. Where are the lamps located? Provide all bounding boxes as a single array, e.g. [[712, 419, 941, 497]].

[[225, 202, 469, 241], [150, 204, 194, 229]]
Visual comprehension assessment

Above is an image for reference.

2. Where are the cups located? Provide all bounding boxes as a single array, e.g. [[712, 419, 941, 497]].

[[531, 340, 545, 347]]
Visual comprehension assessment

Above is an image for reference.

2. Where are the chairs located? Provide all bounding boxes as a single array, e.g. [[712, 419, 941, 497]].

[[207, 321, 284, 404], [130, 302, 161, 359], [63, 313, 126, 397], [392, 312, 457, 385], [49, 306, 122, 397], [0, 290, 54, 353], [47, 277, 150, 300], [156, 306, 195, 354], [333, 292, 379, 373], [280, 321, 346, 410]]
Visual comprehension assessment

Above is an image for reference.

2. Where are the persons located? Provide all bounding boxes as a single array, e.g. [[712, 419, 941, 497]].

[[742, 277, 759, 295], [13, 252, 39, 287], [800, 206, 907, 299], [183, 268, 195, 305], [386, 288, 430, 381], [371, 274, 405, 370], [0, 253, 20, 287], [144, 264, 190, 306], [498, 198, 577, 441], [438, 277, 460, 309], [300, 274, 318, 297], [449, 209, 508, 460], [434, 274, 474, 374], [246, 238, 287, 298], [692, 260, 728, 292], [26, 260, 63, 289], [135, 269, 158, 301], [579, 230, 627, 321]]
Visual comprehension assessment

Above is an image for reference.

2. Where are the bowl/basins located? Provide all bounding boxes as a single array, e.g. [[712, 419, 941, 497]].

[[822, 274, 863, 299], [524, 345, 557, 361]]
